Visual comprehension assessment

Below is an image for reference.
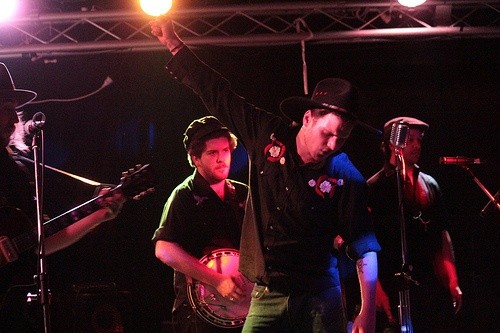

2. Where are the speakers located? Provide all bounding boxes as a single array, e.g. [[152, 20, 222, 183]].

[[73, 290, 135, 333]]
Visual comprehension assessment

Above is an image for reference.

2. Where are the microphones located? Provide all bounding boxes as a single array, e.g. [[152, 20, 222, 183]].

[[390, 122, 410, 172], [24, 119, 46, 135], [439, 157, 480, 165], [480, 191, 500, 218]]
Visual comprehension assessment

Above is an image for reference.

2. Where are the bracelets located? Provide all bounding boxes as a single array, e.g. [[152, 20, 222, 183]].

[[167, 41, 185, 51]]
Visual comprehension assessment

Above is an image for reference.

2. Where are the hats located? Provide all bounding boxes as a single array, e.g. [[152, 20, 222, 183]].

[[184, 116, 223, 151], [280, 78, 384, 135], [0, 62, 37, 107], [384, 117, 429, 130]]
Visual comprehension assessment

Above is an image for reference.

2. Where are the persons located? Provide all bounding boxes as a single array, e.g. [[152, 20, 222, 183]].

[[366, 117, 463, 333], [149, 12, 381, 333], [0, 62, 125, 333], [152, 115, 257, 333]]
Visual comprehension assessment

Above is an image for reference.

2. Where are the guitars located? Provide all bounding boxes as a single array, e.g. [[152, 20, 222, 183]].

[[187, 247, 257, 328], [0, 157, 170, 304]]
